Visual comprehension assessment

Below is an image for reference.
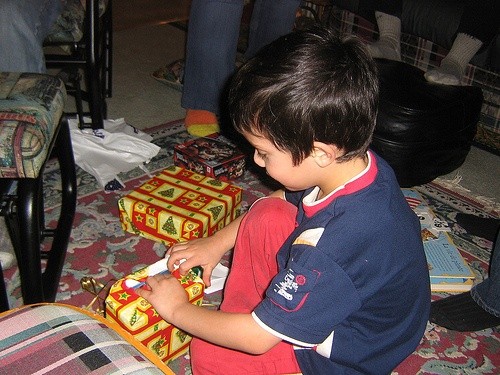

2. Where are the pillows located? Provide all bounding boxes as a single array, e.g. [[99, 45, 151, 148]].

[[152, 58, 186, 92]]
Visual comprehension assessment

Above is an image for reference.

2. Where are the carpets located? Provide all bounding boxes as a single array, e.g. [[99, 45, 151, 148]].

[[0, 118, 500, 375]]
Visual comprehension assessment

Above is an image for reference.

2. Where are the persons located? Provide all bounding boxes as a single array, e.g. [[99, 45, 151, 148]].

[[361, 0, 500, 87], [182, 0, 303, 140], [0, 0, 69, 271], [134, 25, 434, 375], [430, 228, 499, 332]]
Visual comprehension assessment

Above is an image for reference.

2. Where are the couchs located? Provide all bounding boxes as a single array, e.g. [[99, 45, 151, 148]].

[[291, 0, 500, 154]]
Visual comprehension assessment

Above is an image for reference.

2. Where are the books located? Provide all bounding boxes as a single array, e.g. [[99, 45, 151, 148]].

[[398, 185, 476, 293]]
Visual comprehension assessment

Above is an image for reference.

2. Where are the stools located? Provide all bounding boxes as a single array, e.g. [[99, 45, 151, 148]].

[[369, 57, 483, 188]]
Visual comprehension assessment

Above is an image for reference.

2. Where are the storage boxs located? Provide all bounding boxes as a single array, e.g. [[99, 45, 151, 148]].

[[174, 137, 247, 182], [104, 258, 206, 365], [118, 166, 243, 247]]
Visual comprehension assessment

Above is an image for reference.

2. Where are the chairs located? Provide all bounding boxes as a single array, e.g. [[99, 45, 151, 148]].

[[1, 72, 78, 312], [41, 0, 113, 129], [0, 303, 175, 375]]
